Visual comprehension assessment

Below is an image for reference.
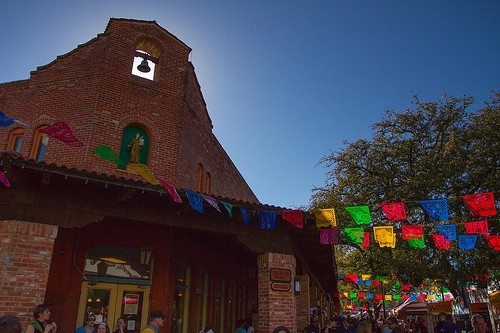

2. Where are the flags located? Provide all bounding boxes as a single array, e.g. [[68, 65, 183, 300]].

[[0, 108, 499, 313]]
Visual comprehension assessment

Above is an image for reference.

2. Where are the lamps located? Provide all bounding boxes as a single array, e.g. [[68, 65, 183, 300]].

[[140, 245, 152, 266]]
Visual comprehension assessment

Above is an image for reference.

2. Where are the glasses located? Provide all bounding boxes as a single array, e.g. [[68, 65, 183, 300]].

[[478, 323, 487, 326], [157, 317, 164, 321], [388, 323, 393, 325], [312, 320, 318, 322], [88, 318, 96, 321]]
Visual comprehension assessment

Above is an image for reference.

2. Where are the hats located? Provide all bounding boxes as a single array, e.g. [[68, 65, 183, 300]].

[[151, 311, 166, 318]]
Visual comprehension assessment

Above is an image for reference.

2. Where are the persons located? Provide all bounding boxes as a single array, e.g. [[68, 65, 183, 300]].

[[0, 301, 493, 333]]
